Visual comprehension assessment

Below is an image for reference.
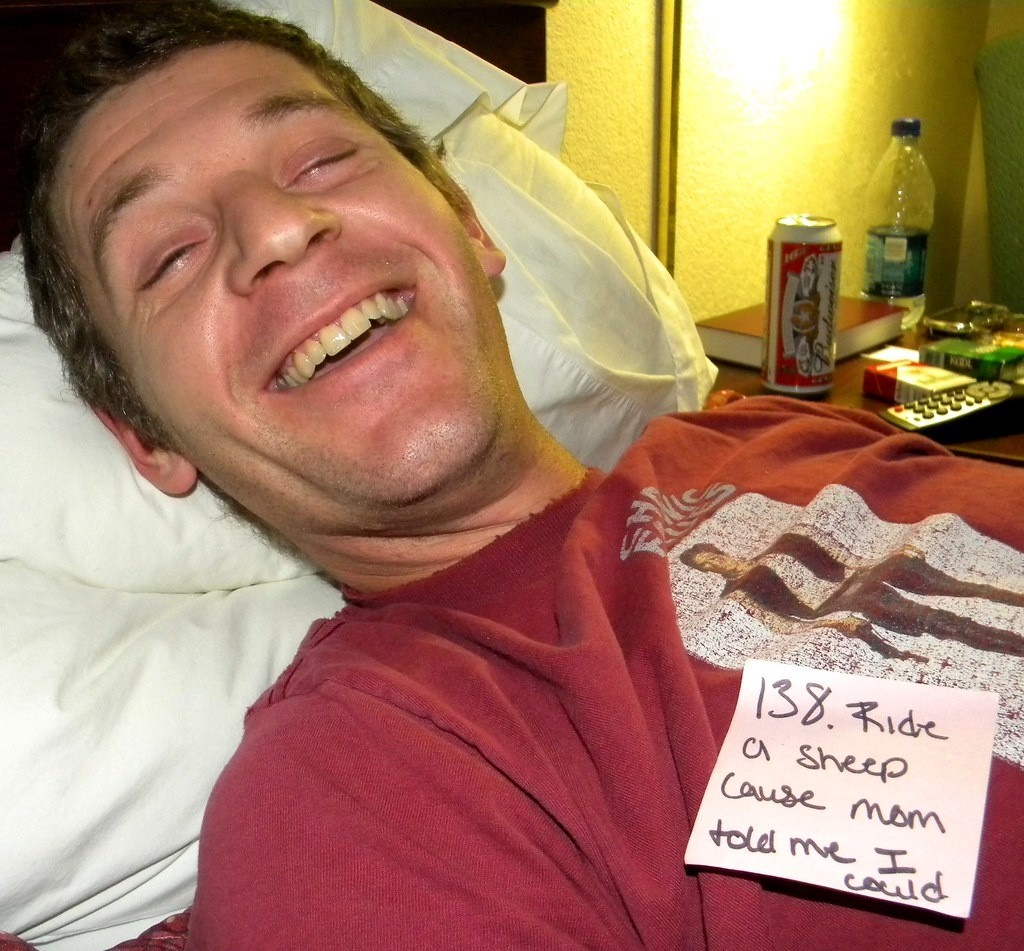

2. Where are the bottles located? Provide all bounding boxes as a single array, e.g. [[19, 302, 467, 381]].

[[856, 119, 935, 331]]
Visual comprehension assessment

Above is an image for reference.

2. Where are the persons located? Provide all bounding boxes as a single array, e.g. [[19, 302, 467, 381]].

[[679, 527, 1024, 665], [1, 0, 1024, 951]]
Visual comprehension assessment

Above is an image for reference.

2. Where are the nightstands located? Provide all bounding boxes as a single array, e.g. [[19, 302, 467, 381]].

[[702, 359, 1024, 474]]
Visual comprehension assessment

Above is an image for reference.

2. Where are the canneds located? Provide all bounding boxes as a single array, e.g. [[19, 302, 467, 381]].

[[762, 215, 843, 394]]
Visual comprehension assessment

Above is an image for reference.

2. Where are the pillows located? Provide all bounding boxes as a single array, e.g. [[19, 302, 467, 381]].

[[0, 0, 719, 950]]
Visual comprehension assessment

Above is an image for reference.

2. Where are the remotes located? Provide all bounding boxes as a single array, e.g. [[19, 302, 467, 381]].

[[877, 375, 1024, 443]]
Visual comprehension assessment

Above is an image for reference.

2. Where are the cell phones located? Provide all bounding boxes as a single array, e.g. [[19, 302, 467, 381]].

[[921, 299, 1009, 334]]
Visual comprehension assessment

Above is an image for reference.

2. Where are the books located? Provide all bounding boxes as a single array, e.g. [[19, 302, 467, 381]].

[[694, 296, 912, 372]]
